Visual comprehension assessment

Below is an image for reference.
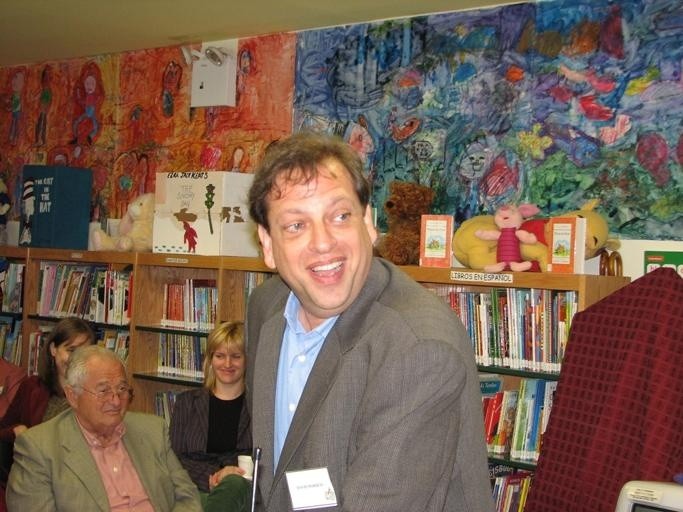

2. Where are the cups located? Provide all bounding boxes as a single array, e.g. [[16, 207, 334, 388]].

[[237, 454, 254, 481]]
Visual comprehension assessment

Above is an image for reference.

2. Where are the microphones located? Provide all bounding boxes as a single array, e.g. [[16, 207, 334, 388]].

[[250, 446, 261, 512]]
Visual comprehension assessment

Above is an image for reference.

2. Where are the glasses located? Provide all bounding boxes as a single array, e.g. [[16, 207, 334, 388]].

[[80, 385, 132, 403]]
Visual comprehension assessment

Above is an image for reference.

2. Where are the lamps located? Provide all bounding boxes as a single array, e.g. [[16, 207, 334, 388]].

[[177, 40, 237, 108]]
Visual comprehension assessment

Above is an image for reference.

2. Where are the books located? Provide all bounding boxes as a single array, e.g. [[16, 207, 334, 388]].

[[0, 257, 133, 377], [422, 283, 578, 512], [244, 271, 277, 296], [152, 277, 218, 422]]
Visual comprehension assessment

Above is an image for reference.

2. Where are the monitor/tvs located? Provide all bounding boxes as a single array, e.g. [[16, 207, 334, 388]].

[[613, 479, 683, 512]]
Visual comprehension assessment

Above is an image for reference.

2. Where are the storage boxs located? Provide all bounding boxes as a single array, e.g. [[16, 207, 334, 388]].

[[152, 171, 260, 256], [18, 164, 93, 251]]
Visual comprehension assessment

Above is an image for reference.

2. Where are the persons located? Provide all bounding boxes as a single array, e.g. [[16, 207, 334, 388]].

[[0, 317, 99, 511], [243, 130, 498, 512], [5, 345, 203, 511], [0, 356, 27, 416], [169, 319, 254, 511]]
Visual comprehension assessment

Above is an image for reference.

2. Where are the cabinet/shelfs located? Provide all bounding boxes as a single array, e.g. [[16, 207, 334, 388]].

[[0, 244, 632, 511]]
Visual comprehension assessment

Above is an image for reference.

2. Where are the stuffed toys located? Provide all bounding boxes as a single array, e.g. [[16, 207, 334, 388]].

[[0, 179, 11, 229], [474, 201, 540, 272], [91, 192, 155, 252], [452, 197, 621, 275], [372, 179, 436, 266]]
